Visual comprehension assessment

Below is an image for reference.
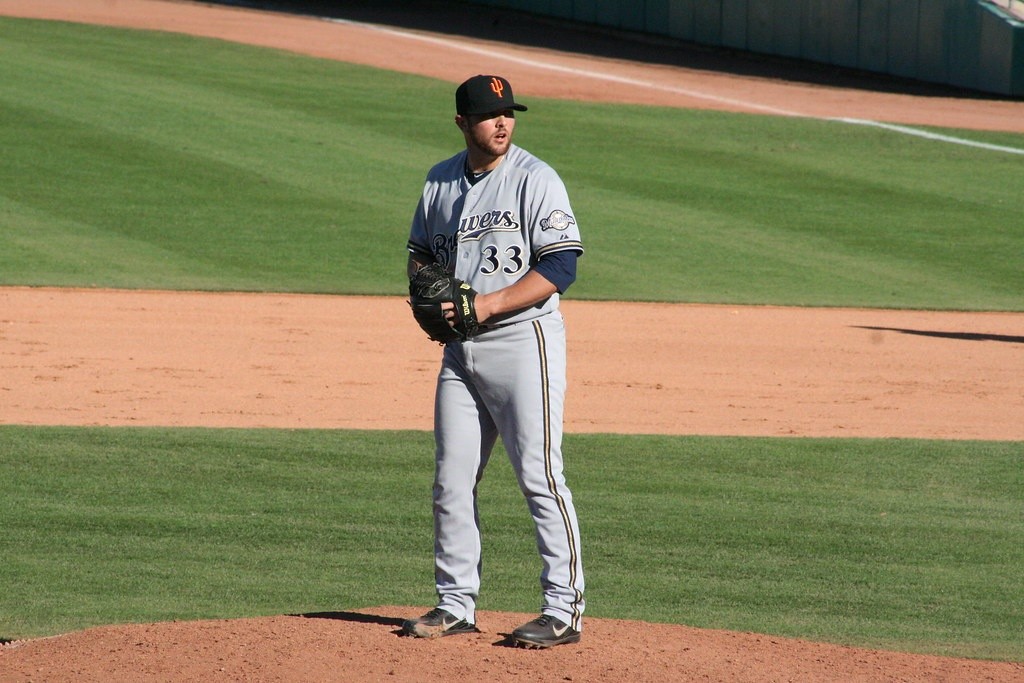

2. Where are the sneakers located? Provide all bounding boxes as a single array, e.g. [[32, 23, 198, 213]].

[[402, 608, 475, 639], [513, 614, 581, 650]]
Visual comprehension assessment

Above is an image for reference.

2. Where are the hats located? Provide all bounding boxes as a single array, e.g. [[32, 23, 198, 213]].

[[456, 74, 527, 115]]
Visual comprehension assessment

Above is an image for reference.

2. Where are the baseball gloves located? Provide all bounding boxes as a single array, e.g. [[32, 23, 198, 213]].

[[404, 262, 483, 348]]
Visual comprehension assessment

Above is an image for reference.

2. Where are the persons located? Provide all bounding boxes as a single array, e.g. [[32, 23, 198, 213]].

[[406, 73, 587, 653]]
